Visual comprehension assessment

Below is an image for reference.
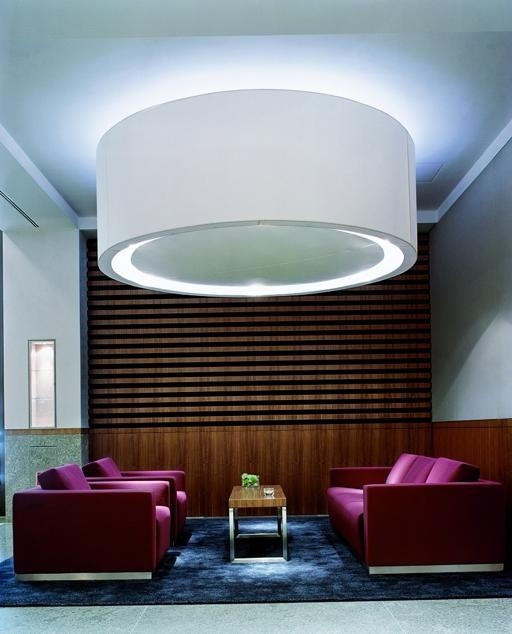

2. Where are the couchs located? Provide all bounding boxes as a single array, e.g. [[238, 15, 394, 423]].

[[327, 453, 504, 574]]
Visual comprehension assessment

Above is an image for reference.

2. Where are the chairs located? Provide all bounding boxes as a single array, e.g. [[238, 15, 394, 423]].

[[13, 463, 173, 580], [81, 456, 188, 546]]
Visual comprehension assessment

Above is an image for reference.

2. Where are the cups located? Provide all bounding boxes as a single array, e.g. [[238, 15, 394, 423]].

[[263, 487, 276, 497]]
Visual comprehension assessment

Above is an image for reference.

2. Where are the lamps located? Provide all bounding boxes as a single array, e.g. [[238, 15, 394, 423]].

[[96, 88, 418, 298]]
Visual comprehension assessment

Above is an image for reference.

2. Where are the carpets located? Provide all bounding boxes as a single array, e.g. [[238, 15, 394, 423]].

[[0, 516, 512, 607]]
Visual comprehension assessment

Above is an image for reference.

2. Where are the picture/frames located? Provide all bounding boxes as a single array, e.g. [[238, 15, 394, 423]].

[[27, 339, 56, 429]]
